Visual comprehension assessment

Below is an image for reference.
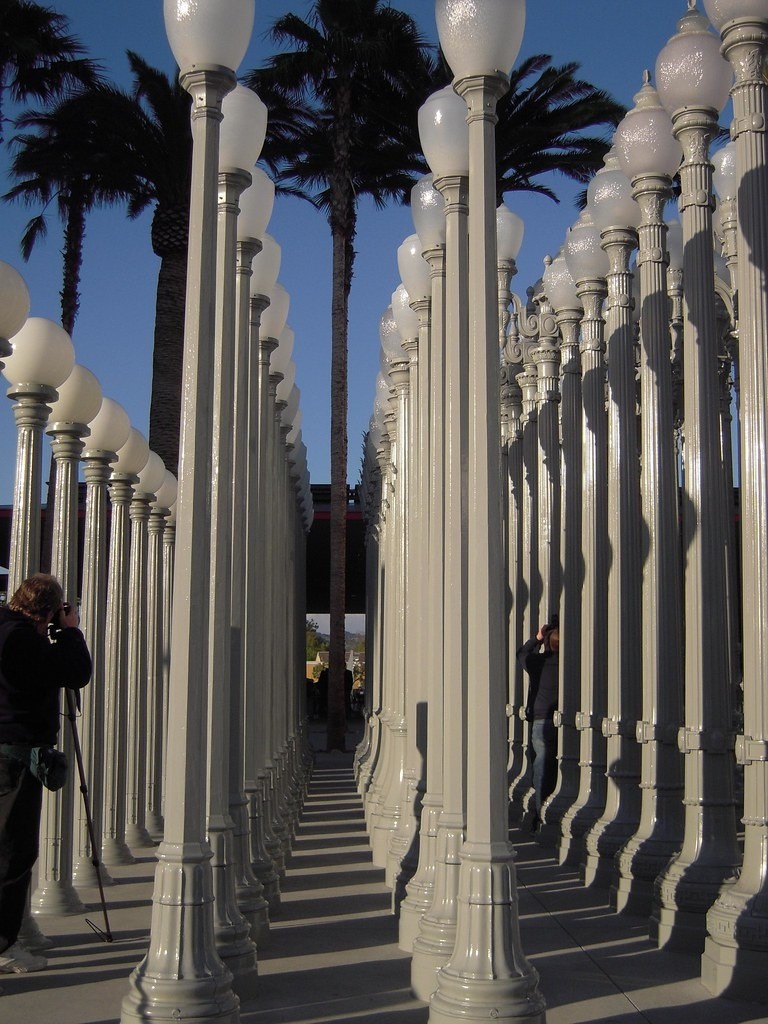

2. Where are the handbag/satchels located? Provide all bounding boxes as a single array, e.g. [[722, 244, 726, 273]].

[[0, 744, 67, 791]]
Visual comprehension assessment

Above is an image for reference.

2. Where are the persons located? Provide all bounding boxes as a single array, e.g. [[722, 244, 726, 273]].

[[0, 572, 92, 972], [319, 659, 353, 747], [516, 623, 559, 837]]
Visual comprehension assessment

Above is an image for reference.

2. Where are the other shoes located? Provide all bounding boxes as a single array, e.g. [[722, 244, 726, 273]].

[[529, 816, 540, 836]]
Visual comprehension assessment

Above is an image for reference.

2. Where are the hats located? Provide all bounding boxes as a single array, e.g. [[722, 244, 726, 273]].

[[546, 614, 559, 631]]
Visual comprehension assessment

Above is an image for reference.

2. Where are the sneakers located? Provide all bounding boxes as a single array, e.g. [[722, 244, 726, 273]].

[[0, 941, 48, 974]]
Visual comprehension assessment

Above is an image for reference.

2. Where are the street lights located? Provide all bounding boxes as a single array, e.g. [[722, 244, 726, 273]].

[[103, 427, 181, 867], [79, 398, 130, 889], [120, 0, 255, 1024], [426, 0, 544, 1024], [354, 73, 525, 1003], [205, 83, 313, 1003], [610, 71, 684, 915], [30, 366, 104, 920], [656, 0, 768, 999], [0, 318, 77, 954], [500, 149, 642, 886]]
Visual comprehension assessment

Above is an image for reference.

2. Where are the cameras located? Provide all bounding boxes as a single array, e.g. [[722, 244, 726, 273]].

[[50, 603, 81, 625]]
